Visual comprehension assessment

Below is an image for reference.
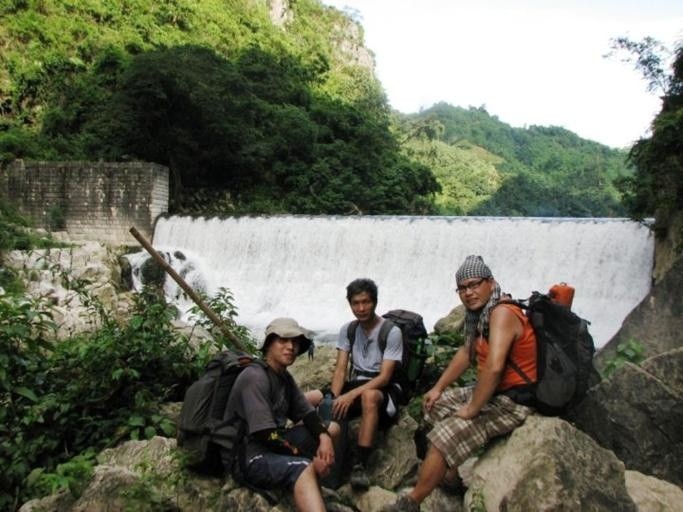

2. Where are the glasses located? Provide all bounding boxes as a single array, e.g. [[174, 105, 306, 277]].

[[454, 278, 485, 294]]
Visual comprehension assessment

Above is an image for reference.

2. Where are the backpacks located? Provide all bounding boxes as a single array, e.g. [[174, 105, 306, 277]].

[[485, 290, 596, 415], [176, 350, 294, 479], [347, 310, 428, 400]]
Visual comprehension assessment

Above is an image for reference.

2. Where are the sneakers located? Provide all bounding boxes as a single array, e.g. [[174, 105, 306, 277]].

[[351, 464, 370, 489], [382, 496, 420, 512]]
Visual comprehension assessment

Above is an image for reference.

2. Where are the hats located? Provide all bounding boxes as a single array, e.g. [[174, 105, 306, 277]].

[[255, 318, 311, 355]]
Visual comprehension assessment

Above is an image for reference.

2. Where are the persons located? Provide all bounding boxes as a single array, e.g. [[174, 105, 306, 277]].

[[223, 317, 342, 512], [303, 278, 405, 490], [382, 255, 539, 512]]
